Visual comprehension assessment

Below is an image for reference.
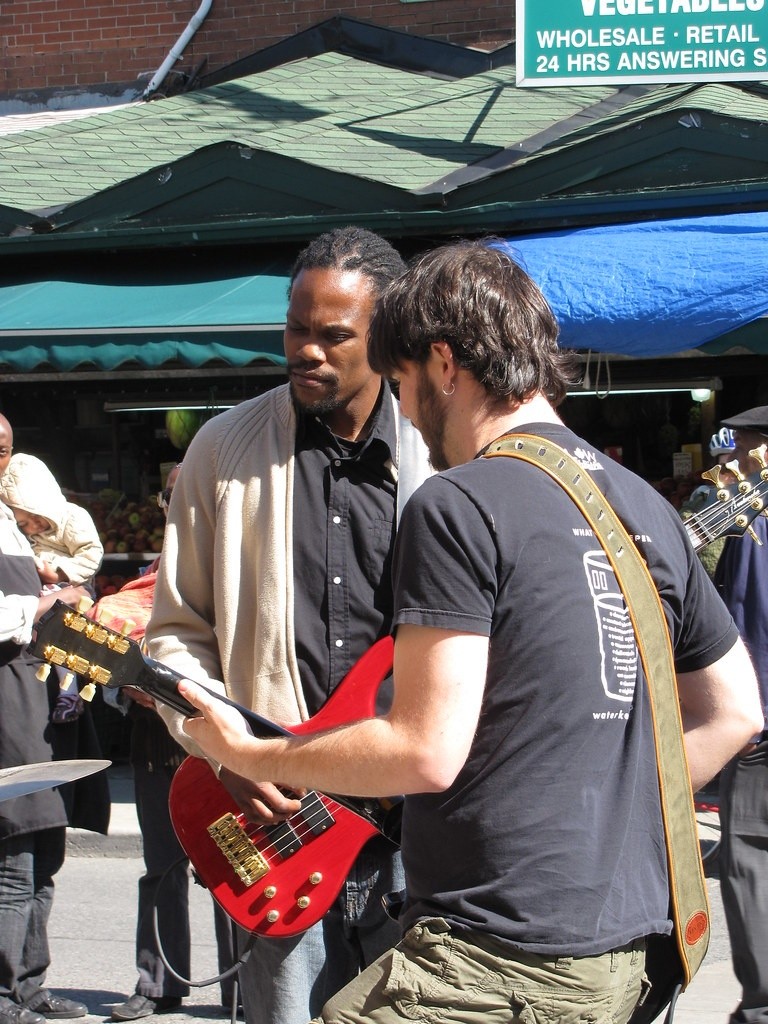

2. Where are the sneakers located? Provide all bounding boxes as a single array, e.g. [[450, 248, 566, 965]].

[[111, 994, 181, 1020], [33, 995, 87, 1018], [223, 1004, 244, 1020], [52, 693, 85, 723], [0, 1003, 46, 1024]]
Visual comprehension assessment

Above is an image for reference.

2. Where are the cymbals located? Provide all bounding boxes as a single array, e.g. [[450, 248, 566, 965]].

[[0, 756, 113, 803]]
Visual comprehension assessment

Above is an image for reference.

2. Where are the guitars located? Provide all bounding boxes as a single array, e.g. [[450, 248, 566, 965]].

[[157, 437, 768, 952], [33, 585, 445, 862]]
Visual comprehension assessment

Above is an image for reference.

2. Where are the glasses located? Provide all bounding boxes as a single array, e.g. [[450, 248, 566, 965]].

[[157, 489, 172, 508], [720, 462, 731, 474]]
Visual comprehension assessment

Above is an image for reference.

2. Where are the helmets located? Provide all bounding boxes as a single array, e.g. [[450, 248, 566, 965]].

[[710, 427, 738, 457]]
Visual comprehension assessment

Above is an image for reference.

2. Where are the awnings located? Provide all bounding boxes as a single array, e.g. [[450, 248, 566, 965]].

[[0, 211, 766, 369]]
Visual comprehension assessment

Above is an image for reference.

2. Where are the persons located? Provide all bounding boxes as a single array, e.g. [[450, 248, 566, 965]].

[[145, 226, 443, 1024], [179, 234, 765, 1024], [0, 414, 244, 1024], [677, 406, 768, 1024]]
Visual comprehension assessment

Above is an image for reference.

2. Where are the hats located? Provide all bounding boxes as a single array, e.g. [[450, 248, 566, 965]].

[[720, 406, 768, 431]]
